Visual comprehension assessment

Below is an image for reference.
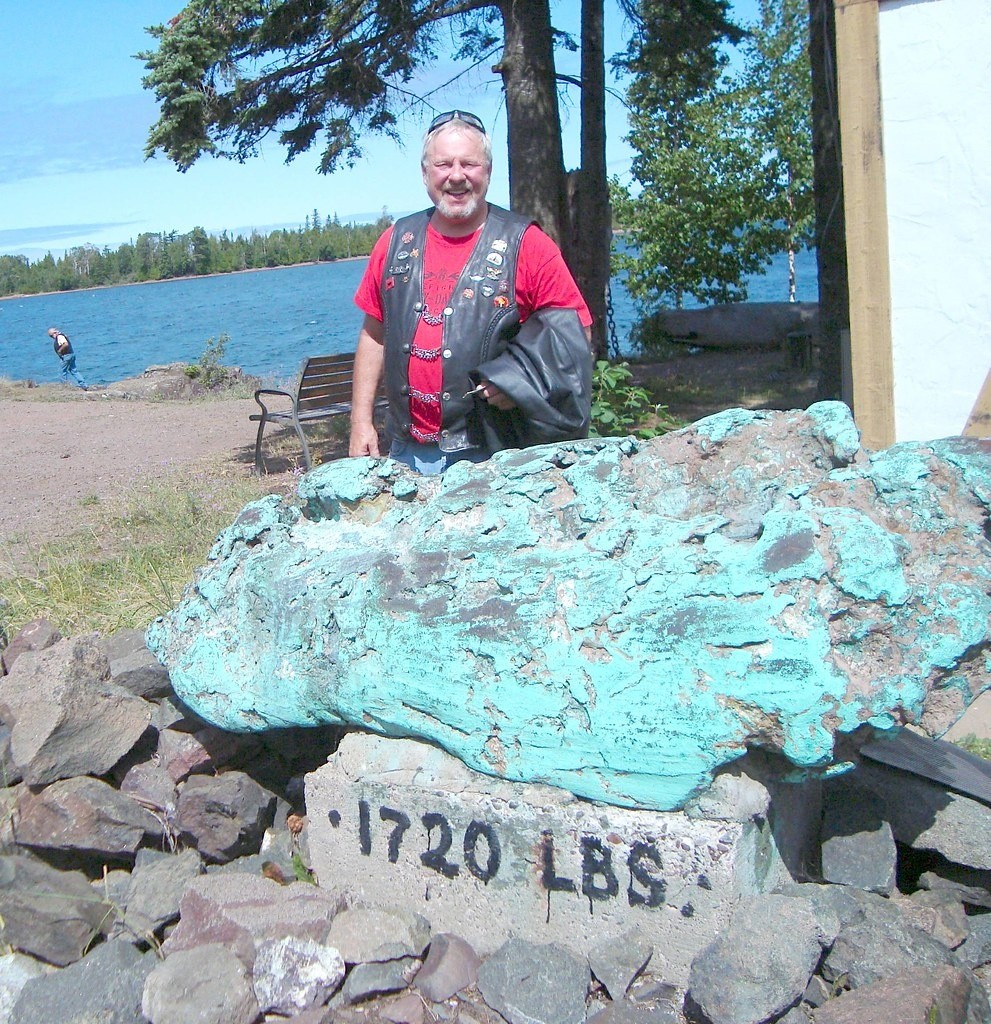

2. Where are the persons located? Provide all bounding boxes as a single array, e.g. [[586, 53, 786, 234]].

[[48, 328, 89, 391], [349, 110, 592, 474]]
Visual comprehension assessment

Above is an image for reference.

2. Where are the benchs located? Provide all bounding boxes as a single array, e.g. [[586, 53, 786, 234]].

[[248, 352, 391, 478]]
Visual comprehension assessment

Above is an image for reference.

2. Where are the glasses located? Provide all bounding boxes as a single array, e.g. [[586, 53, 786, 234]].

[[428, 110, 486, 134]]
